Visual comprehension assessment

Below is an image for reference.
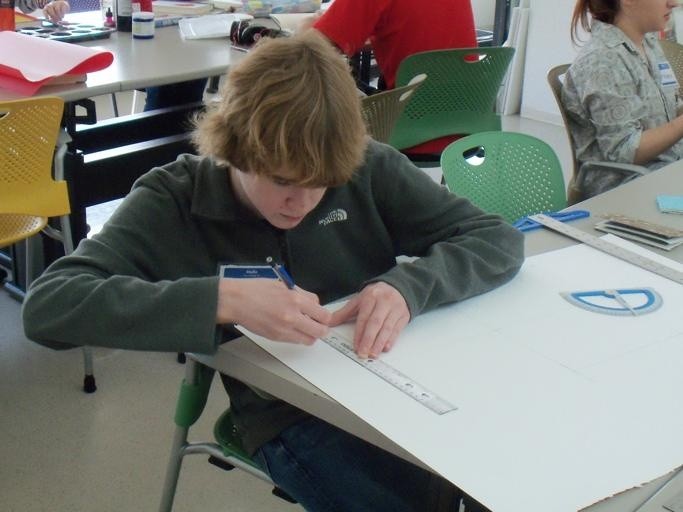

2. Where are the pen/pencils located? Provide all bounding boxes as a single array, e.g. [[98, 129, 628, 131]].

[[266, 256, 305, 294]]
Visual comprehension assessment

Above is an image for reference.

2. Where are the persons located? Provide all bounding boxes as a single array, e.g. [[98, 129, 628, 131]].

[[19, 32, 527, 512], [556, 0, 681, 209], [301, 1, 483, 158], [13, 0, 70, 22]]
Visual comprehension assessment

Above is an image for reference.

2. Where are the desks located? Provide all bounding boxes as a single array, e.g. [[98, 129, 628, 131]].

[[1, 10, 320, 269], [185, 156, 683, 512]]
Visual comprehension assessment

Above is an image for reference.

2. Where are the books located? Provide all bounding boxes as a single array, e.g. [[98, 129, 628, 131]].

[[592, 213, 682, 253]]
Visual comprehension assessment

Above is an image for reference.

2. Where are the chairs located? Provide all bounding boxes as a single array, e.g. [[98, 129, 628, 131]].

[[0, 97, 99, 393], [157, 352, 310, 512]]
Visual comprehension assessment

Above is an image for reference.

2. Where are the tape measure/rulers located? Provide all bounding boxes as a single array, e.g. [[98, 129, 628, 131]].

[[319, 329, 457, 415], [529, 214, 683, 283], [559, 287, 663, 316]]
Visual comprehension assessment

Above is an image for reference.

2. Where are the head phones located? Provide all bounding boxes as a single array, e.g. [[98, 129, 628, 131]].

[[230, 14, 282, 47]]
